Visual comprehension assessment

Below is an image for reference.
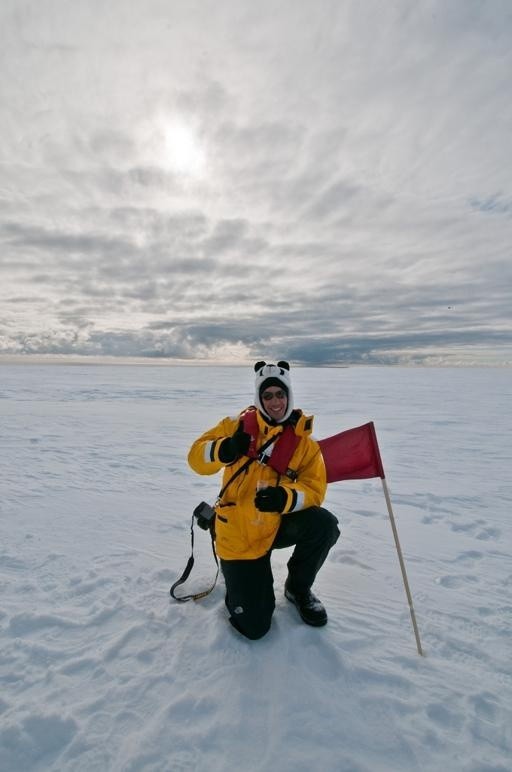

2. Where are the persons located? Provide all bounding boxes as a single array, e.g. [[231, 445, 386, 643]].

[[185, 360, 339, 641]]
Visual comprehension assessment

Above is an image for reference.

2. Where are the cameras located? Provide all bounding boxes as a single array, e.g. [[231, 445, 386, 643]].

[[194, 502, 214, 529]]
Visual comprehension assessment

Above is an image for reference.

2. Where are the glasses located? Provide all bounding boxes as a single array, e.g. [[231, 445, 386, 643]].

[[263, 389, 286, 399]]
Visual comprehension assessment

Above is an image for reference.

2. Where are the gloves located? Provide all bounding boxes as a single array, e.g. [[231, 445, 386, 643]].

[[220, 420, 250, 457], [255, 486, 288, 513]]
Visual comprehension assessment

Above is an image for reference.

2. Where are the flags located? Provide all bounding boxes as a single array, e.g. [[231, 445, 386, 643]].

[[318, 419, 387, 485]]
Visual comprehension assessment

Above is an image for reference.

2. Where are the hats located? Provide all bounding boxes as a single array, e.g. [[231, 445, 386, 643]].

[[254, 361, 294, 423]]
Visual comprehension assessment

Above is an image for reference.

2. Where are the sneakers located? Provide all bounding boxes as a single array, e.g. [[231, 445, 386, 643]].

[[285, 580, 327, 626]]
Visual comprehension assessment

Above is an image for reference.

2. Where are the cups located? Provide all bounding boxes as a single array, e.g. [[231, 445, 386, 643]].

[[256, 479, 269, 491]]
